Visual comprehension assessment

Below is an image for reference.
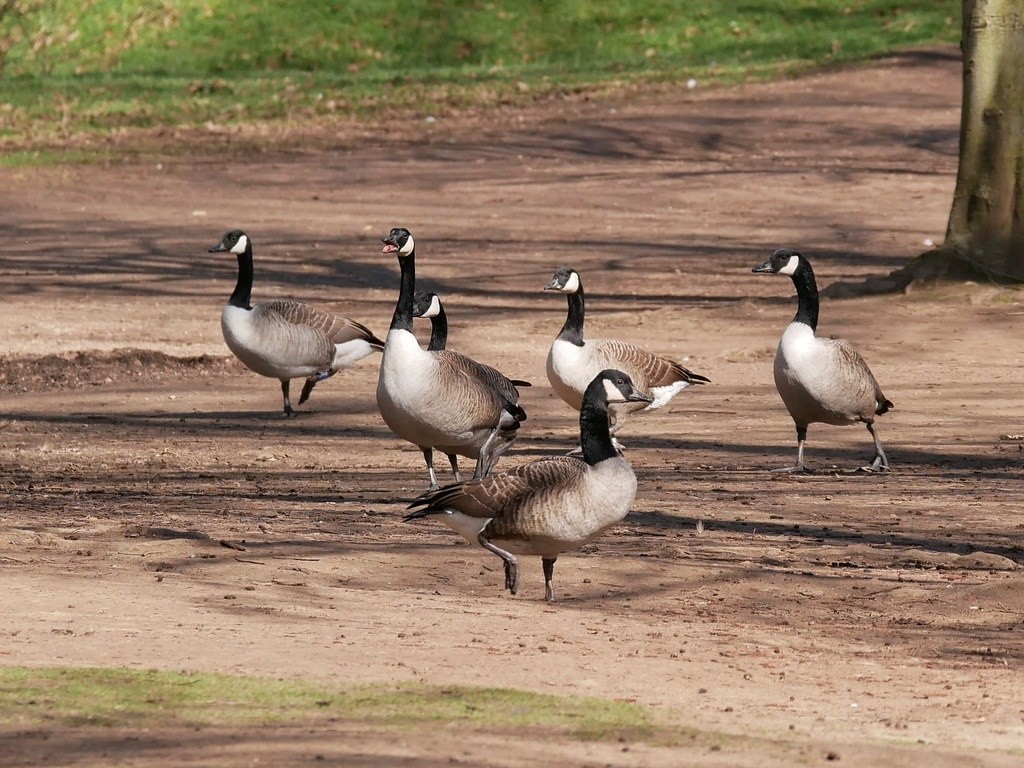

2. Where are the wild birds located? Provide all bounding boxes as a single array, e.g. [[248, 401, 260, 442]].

[[751, 247, 896, 473], [208, 226, 712, 600]]
